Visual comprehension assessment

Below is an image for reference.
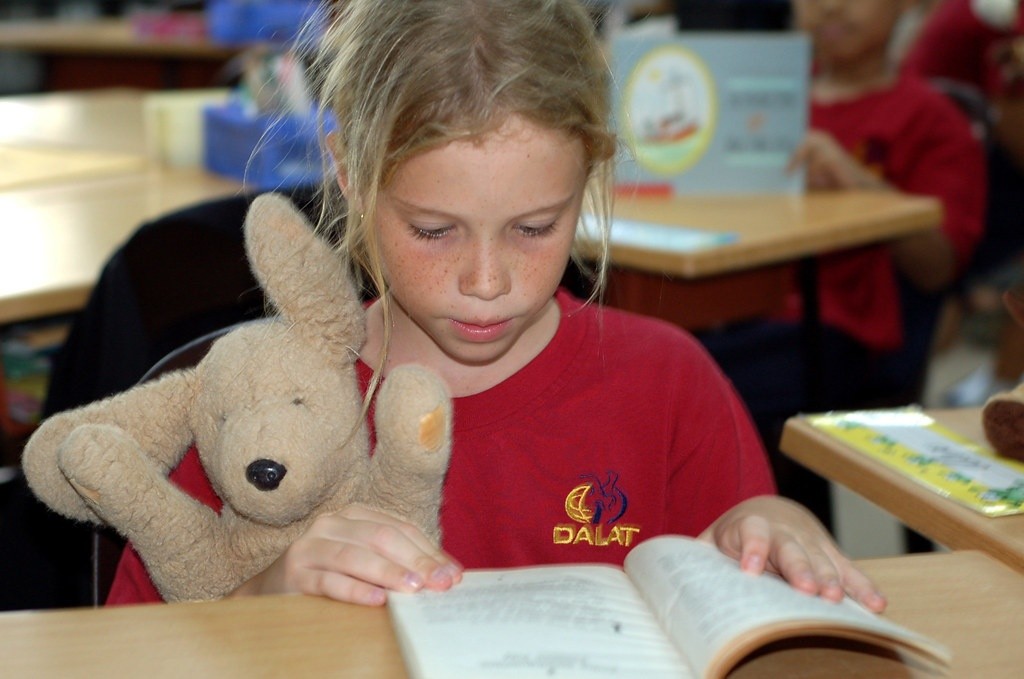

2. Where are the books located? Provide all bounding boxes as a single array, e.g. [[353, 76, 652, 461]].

[[385, 534, 951, 678]]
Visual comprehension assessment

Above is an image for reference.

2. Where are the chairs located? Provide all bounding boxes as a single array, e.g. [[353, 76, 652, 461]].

[[37, 185, 597, 606]]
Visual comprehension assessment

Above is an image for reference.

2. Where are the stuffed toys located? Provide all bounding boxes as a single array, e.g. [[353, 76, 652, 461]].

[[22, 193, 456, 606]]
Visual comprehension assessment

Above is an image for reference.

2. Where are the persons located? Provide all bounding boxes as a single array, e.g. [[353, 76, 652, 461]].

[[689, 1, 985, 534], [106, 1, 880, 609]]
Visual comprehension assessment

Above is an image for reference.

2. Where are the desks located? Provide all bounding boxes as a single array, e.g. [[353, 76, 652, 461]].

[[0, 20, 220, 55], [0, 89, 257, 321], [575, 190, 943, 529], [779, 408, 1024, 576], [0, 549, 1024, 678]]
